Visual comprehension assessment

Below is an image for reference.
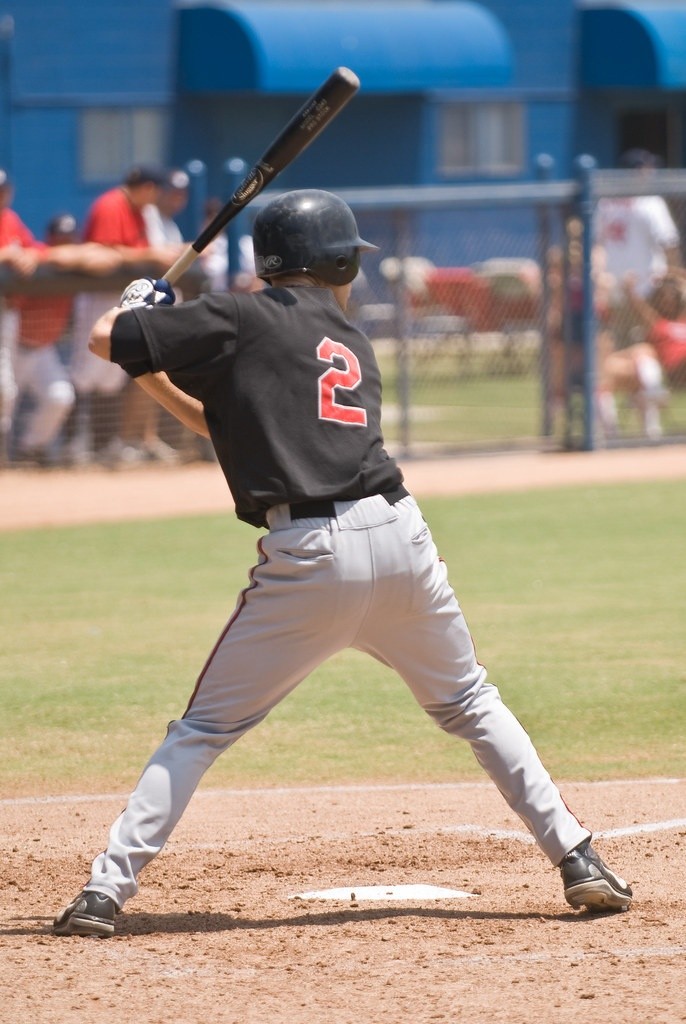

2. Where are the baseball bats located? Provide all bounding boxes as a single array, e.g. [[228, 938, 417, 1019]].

[[152, 64, 362, 291]]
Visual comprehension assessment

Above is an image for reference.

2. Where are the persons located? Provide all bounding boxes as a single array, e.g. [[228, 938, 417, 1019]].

[[0, 162, 686, 467], [51, 189, 631, 936]]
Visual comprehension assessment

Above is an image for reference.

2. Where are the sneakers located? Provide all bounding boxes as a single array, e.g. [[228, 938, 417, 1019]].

[[559, 841, 632, 908], [50, 891, 117, 937]]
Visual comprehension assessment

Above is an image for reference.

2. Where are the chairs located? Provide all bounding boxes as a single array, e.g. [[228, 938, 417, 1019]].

[[390, 254, 545, 373]]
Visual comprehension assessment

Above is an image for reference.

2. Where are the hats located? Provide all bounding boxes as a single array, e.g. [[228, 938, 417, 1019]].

[[125, 164, 169, 187], [0, 170, 13, 183], [161, 168, 189, 187], [46, 215, 73, 233]]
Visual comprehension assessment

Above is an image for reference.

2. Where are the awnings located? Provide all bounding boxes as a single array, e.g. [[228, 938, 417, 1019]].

[[173, 1, 519, 98], [578, 8, 686, 90]]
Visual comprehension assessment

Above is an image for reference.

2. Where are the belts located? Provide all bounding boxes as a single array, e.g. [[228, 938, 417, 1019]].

[[265, 482, 409, 530]]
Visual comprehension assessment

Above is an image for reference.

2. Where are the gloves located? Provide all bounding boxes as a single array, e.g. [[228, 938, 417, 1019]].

[[119, 276, 177, 313]]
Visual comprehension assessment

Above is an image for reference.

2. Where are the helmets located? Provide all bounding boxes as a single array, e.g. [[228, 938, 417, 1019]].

[[252, 189, 382, 286]]
